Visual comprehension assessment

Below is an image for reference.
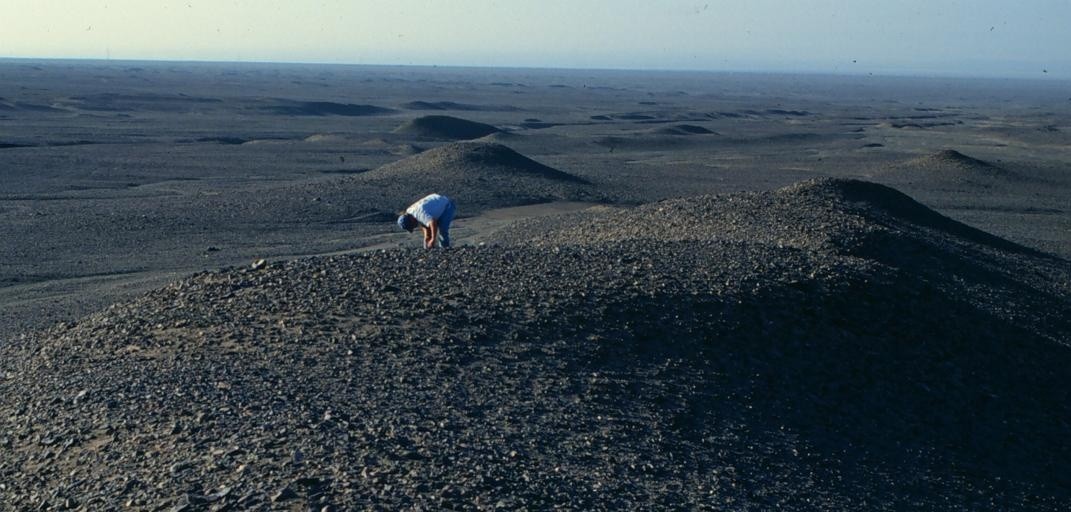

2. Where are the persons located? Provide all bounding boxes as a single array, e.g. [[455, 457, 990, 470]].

[[397, 194, 457, 250]]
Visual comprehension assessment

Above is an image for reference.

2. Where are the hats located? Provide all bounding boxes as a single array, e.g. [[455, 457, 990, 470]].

[[398, 215, 415, 234]]
